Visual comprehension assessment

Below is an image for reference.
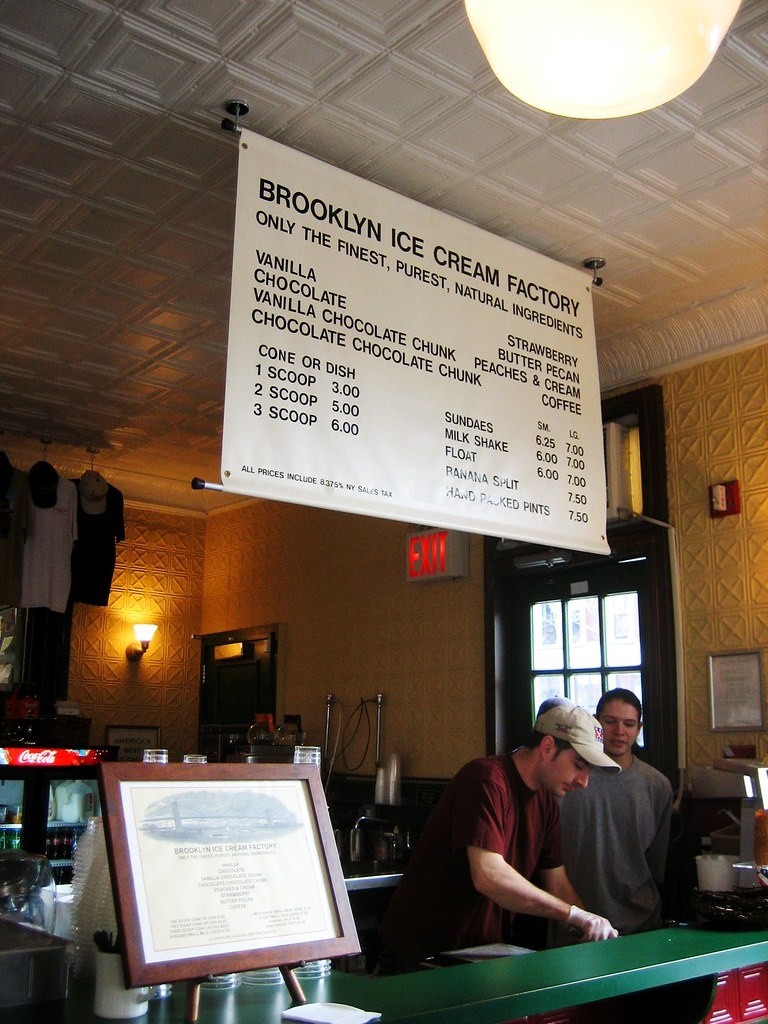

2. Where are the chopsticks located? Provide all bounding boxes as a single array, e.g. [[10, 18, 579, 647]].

[[93, 930, 120, 953]]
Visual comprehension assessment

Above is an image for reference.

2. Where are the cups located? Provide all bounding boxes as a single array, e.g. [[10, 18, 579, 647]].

[[93, 949, 148, 1019], [0, 806, 11, 824], [148, 958, 332, 1000], [42, 816, 121, 984], [375, 752, 401, 806], [12, 806, 23, 823], [143, 749, 209, 765]]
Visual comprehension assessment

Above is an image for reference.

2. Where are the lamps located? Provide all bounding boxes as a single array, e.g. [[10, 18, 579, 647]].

[[126, 624, 159, 662], [464, 0, 743, 120]]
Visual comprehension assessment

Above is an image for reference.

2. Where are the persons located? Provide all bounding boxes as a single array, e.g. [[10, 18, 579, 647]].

[[538, 695, 573, 717], [368, 705, 619, 979], [556, 688, 673, 940]]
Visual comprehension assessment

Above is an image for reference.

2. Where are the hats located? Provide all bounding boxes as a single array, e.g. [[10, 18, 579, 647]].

[[79, 470, 107, 514], [535, 697, 621, 777], [28, 461, 59, 509]]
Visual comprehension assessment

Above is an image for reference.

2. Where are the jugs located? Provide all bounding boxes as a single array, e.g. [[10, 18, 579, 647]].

[[275, 714, 304, 746], [247, 714, 275, 745]]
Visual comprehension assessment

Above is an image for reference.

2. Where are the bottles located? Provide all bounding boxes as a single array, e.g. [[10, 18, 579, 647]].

[[49, 780, 94, 820], [0, 828, 80, 885]]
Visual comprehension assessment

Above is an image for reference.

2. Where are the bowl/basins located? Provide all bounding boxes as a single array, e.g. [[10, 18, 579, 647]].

[[696, 855, 739, 891]]
